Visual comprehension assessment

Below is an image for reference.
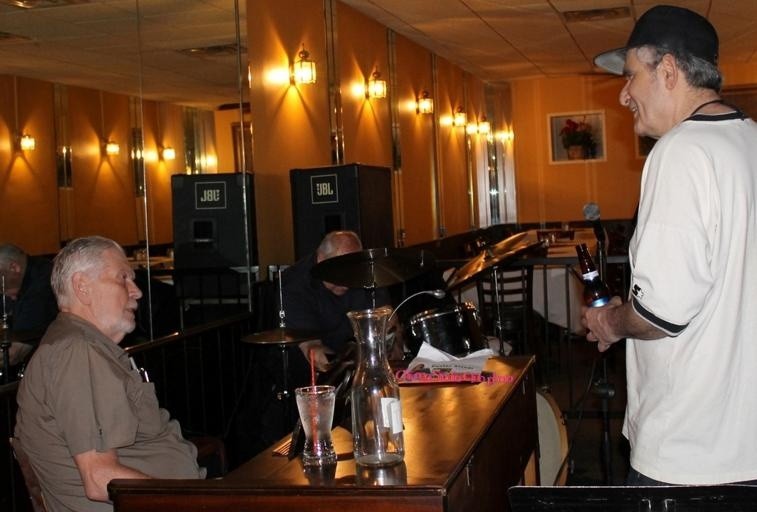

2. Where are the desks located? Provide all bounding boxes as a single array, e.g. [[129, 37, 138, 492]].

[[128, 257, 174, 276], [107, 355, 540, 512]]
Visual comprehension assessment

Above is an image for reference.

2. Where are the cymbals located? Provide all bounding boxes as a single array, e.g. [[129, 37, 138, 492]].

[[309, 246, 438, 291], [444, 229, 546, 289], [236, 327, 329, 348]]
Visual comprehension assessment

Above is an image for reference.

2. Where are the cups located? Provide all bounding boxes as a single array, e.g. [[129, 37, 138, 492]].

[[295, 386, 339, 465], [135, 247, 150, 263]]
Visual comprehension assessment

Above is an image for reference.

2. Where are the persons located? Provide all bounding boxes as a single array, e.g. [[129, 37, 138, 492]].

[[9, 235, 206, 511], [0, 243, 58, 377], [577, 3, 755, 487], [275, 229, 387, 376]]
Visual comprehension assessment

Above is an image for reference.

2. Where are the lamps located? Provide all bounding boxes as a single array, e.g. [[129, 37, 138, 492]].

[[11, 133, 177, 162], [288, 42, 490, 136]]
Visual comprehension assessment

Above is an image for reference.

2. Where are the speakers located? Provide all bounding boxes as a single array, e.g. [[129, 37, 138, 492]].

[[290, 163, 396, 264], [171, 173, 257, 301]]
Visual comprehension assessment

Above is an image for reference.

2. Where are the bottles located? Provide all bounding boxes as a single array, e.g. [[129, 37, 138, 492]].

[[347, 308, 405, 468], [575, 242, 610, 312]]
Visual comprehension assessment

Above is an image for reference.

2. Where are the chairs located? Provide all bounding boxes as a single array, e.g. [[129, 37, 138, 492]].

[[456, 222, 624, 380]]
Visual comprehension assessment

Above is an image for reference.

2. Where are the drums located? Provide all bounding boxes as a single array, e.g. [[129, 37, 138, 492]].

[[521, 381, 570, 486], [401, 301, 488, 359]]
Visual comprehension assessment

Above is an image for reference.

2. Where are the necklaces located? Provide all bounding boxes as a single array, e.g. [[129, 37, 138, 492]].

[[683, 93, 735, 117]]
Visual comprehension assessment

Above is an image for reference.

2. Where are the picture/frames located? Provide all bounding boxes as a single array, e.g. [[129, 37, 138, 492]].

[[634, 129, 658, 160], [547, 109, 608, 164]]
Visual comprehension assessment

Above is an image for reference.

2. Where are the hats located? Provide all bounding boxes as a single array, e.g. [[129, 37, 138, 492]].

[[593, 6, 719, 75]]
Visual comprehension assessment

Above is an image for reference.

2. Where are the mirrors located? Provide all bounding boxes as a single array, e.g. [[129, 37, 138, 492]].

[[0, 0, 255, 386]]
